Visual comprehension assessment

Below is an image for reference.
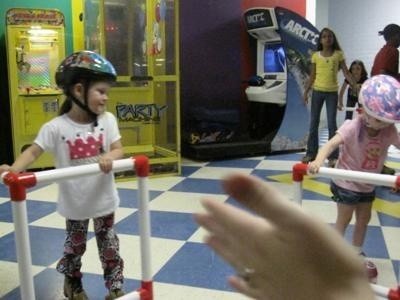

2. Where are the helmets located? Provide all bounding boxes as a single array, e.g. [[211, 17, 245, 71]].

[[55, 49, 118, 89], [357, 72, 400, 125]]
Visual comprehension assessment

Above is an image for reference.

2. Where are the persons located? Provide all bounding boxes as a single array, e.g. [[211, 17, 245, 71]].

[[1, 50, 123, 299], [337, 60, 369, 120], [306, 75, 400, 277], [371, 24, 400, 81], [194, 173, 378, 300], [301, 28, 357, 167]]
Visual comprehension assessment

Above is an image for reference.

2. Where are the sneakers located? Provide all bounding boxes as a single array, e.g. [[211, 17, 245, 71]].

[[359, 251, 378, 280], [328, 158, 336, 168], [302, 155, 313, 164], [63, 275, 88, 300], [105, 287, 125, 300]]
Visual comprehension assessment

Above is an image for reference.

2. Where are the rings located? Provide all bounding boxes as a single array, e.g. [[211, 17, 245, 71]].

[[238, 269, 255, 281]]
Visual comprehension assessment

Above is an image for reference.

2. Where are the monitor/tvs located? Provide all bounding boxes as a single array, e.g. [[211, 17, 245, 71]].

[[264, 40, 285, 72]]
